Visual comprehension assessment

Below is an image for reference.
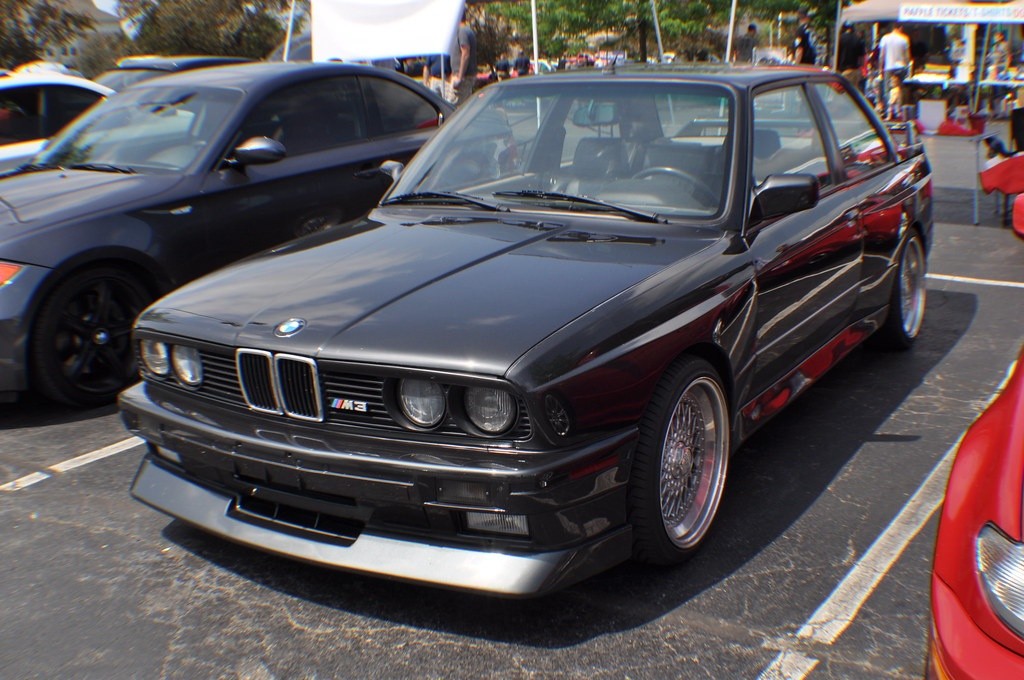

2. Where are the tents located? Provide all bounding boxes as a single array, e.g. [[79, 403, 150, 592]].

[[833, 0, 1024, 114]]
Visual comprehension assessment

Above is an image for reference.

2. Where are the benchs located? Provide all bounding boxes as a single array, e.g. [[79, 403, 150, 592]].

[[639, 142, 819, 193]]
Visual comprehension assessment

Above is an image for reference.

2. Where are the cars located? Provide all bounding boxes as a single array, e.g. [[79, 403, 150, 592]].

[[0, 61, 516, 409], [924, 341, 1024, 680], [113, 61, 937, 603], [0, 56, 354, 177]]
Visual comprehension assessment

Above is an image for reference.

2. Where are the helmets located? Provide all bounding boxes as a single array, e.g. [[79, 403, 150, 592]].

[[845, 19, 855, 28]]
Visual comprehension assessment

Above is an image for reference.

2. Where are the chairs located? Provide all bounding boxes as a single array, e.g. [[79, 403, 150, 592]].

[[573, 137, 629, 178], [692, 130, 780, 208], [278, 102, 335, 153]]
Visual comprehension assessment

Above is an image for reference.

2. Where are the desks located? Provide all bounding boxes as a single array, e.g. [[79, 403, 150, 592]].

[[903, 76, 973, 116], [980, 80, 1023, 116]]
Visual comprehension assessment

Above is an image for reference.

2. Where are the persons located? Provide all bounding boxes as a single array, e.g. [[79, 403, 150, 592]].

[[942, 32, 966, 78], [731, 24, 759, 67], [880, 23, 910, 116], [493, 53, 510, 81], [985, 28, 1012, 125], [405, 8, 477, 109], [513, 48, 531, 77], [837, 24, 867, 70], [544, 51, 603, 72], [866, 62, 882, 112], [841, 68, 859, 88], [786, 5, 818, 66], [884, 73, 905, 121]]
